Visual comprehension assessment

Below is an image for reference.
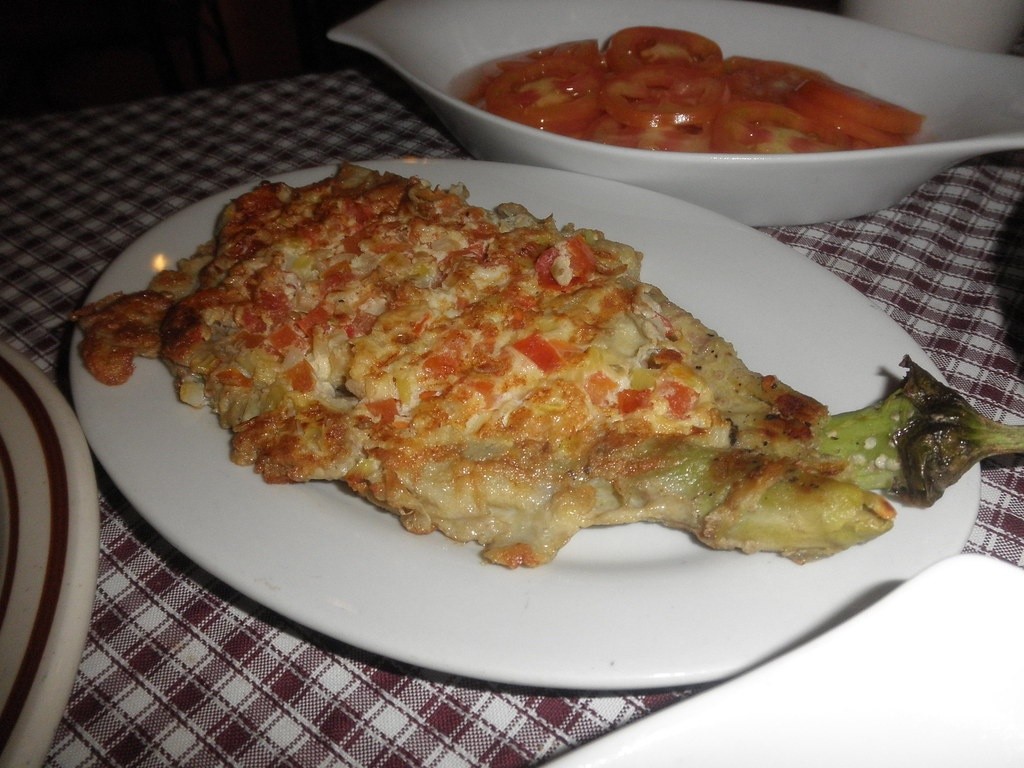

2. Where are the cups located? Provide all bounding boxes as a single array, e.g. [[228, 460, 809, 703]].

[[838, 0, 1024, 57]]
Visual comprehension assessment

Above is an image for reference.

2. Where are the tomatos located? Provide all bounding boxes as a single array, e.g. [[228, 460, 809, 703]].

[[468, 27, 926, 155]]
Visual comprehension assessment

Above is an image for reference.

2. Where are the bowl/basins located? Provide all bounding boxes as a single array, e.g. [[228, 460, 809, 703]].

[[328, 0, 1024, 228]]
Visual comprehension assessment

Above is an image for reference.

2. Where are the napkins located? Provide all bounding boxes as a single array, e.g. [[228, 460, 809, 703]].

[[548, 552, 1024, 768]]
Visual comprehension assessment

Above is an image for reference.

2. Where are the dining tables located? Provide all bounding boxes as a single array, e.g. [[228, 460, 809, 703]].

[[1, 68, 1024, 768]]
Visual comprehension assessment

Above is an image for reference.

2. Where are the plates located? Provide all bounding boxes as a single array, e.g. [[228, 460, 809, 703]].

[[69, 157, 981, 692], [0, 344, 102, 768], [531, 552, 1024, 768]]
